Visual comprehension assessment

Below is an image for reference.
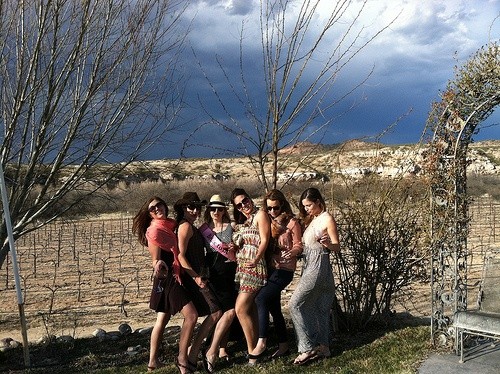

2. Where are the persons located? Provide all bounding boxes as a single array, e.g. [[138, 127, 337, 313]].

[[132, 196, 192, 374], [176, 192, 221, 374], [246, 189, 302, 359], [229, 189, 271, 366], [288, 188, 341, 365], [199, 195, 240, 372]]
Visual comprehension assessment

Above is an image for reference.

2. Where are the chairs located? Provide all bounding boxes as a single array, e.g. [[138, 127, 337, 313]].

[[453, 244, 500, 366]]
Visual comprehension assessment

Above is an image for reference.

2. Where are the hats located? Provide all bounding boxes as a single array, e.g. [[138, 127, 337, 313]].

[[174, 192, 207, 212], [205, 195, 229, 210]]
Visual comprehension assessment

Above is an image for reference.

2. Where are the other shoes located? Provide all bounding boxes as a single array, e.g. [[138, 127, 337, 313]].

[[268, 348, 291, 359], [245, 347, 268, 359], [245, 359, 261, 366]]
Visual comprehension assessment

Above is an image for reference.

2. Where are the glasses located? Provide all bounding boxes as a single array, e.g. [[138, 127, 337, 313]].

[[266, 206, 280, 211], [148, 202, 163, 212], [187, 205, 201, 210], [235, 197, 250, 209], [210, 208, 223, 212]]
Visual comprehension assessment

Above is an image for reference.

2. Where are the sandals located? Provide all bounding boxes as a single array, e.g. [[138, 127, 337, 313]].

[[293, 350, 319, 365], [174, 345, 230, 374], [317, 352, 330, 357], [148, 357, 169, 370]]
